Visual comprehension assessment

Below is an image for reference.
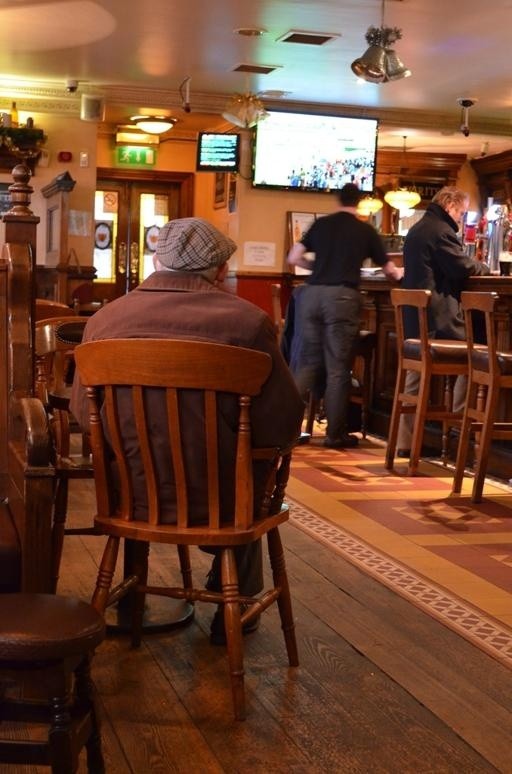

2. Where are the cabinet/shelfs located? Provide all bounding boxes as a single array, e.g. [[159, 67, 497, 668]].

[[469, 147, 511, 226], [374, 147, 468, 231]]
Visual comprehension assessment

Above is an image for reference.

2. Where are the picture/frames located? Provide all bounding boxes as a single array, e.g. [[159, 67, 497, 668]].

[[211, 167, 229, 212], [285, 209, 316, 275]]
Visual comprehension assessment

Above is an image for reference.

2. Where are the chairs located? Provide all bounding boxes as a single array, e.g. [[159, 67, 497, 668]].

[[74, 338, 299, 722], [385, 290, 511, 504], [0, 594, 105, 773], [273, 283, 375, 439], [33, 298, 107, 593]]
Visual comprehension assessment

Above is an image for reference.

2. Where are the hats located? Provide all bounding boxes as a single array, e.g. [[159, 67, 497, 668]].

[[155, 216, 237, 270]]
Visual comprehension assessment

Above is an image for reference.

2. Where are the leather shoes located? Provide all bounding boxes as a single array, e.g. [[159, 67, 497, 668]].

[[396, 447, 427, 457]]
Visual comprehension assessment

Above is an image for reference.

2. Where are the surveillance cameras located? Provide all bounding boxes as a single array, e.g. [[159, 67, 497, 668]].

[[66, 80, 79, 93]]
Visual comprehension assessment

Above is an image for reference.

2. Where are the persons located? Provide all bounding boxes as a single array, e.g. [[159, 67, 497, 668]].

[[71, 216, 305, 643], [288, 181, 403, 446], [394, 188, 491, 457]]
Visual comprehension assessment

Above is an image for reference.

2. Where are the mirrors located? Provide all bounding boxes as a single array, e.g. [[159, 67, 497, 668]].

[[398, 210, 426, 235]]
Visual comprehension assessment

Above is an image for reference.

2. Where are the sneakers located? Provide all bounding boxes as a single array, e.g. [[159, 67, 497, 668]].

[[211, 602, 264, 647], [324, 435, 360, 447], [204, 565, 242, 592]]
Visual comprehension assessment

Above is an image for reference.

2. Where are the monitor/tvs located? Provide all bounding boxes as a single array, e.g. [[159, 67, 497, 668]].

[[251, 107, 380, 194], [196, 132, 240, 172]]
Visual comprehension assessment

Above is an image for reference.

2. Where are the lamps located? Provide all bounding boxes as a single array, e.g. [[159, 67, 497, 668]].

[[383, 133, 422, 210], [129, 112, 180, 136], [355, 183, 385, 216], [79, 90, 108, 125], [222, 69, 271, 131], [350, 0, 412, 86]]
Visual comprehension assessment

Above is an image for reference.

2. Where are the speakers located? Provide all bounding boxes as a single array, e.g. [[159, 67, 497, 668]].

[[80, 94, 105, 122]]
